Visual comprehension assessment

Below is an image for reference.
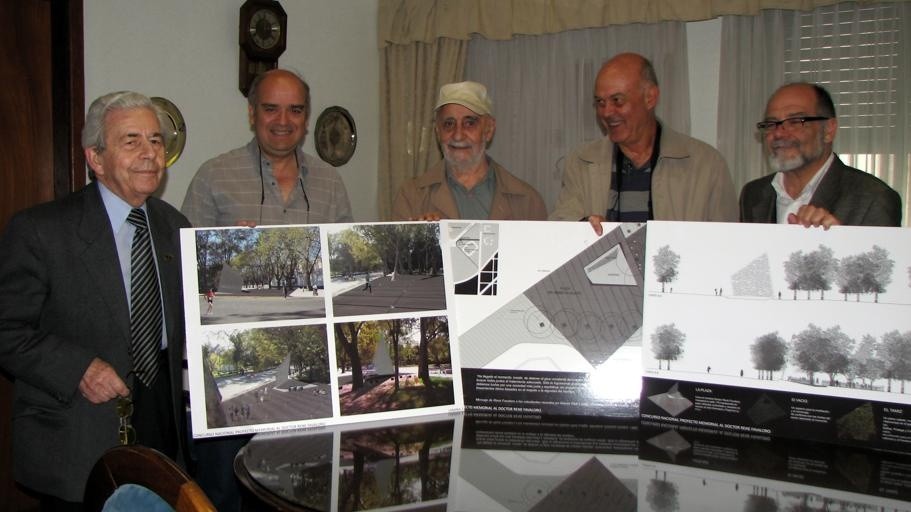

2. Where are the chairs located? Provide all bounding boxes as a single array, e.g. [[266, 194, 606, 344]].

[[79, 443, 219, 512]]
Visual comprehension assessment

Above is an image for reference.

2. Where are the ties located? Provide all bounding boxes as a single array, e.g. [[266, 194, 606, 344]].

[[126, 205, 162, 389]]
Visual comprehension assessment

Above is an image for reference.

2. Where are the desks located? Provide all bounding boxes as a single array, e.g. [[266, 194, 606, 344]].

[[228, 409, 911, 511]]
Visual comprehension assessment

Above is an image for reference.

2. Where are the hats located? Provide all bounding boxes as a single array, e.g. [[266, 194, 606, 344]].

[[430, 79, 497, 119]]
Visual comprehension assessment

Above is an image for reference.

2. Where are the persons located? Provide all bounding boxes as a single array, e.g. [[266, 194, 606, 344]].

[[227, 366, 320, 424], [311, 283, 318, 295], [344, 272, 357, 282], [393, 83, 548, 222], [739, 81, 902, 231], [1, 92, 197, 512], [182, 70, 356, 229], [205, 288, 216, 317], [550, 52, 738, 236], [362, 272, 372, 295]]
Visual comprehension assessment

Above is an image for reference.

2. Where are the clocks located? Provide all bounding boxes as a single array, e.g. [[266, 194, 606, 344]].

[[237, 1, 289, 100]]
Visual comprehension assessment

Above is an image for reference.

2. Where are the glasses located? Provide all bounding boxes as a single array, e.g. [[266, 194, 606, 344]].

[[755, 112, 832, 133]]
